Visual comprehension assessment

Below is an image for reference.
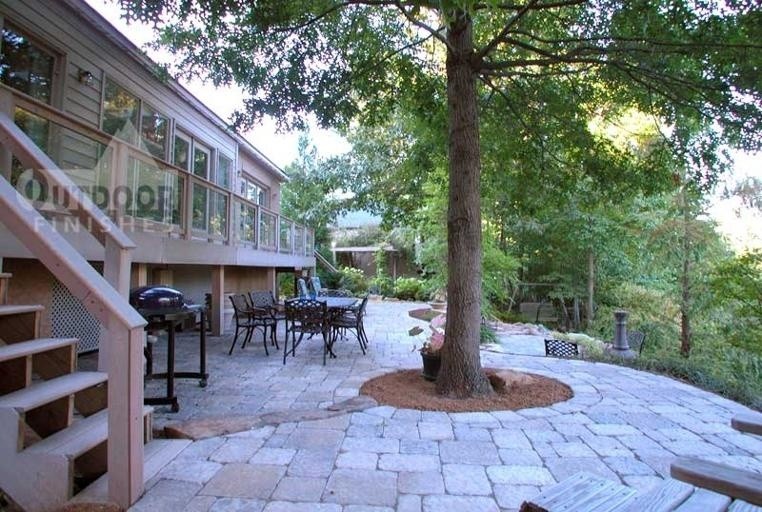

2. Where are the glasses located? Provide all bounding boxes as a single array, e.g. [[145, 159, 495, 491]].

[[79, 70, 94, 87]]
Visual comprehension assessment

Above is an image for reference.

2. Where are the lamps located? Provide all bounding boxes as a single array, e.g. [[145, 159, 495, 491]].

[[228, 287, 369, 366]]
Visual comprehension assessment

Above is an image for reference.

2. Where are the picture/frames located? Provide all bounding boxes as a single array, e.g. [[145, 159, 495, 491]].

[[137, 306, 208, 412]]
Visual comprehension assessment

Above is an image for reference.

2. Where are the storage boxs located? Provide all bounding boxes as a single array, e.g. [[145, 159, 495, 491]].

[[544, 338, 583, 359], [520, 302, 559, 322], [604, 331, 645, 356]]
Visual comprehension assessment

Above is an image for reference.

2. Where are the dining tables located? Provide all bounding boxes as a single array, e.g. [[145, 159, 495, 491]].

[[410, 313, 446, 382]]
